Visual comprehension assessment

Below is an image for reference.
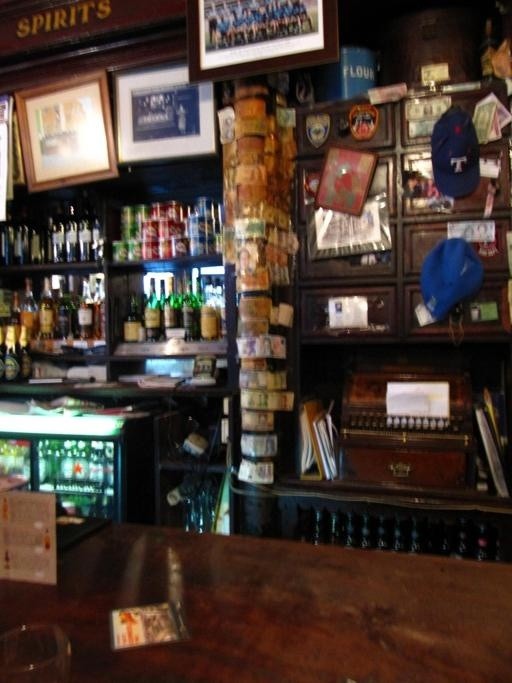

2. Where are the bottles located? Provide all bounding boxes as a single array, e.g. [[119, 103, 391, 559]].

[[123, 273, 223, 343], [39, 440, 116, 518], [0, 276, 104, 382], [312, 506, 511, 563], [0, 204, 102, 263]]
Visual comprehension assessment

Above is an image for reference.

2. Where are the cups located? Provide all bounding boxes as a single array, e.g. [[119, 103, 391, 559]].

[[0, 621, 74, 683]]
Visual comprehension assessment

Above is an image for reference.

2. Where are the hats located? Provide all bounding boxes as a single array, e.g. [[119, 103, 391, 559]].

[[431, 111, 479, 197], [420, 238, 483, 319]]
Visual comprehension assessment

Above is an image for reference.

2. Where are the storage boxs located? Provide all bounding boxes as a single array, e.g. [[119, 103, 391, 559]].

[[332, 429, 471, 497]]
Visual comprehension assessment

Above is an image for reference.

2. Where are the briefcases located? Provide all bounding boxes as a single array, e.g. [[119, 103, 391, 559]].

[[339, 368, 471, 491]]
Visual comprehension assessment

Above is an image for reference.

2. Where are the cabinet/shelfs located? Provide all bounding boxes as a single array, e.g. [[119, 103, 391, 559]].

[[292, 76, 512, 343], [0, 153, 236, 535]]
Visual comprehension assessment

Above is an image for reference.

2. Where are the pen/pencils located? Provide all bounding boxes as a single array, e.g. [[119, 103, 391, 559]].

[[55, 515, 85, 526]]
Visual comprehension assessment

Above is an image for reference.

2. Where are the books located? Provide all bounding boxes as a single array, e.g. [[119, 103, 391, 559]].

[[295, 394, 341, 483]]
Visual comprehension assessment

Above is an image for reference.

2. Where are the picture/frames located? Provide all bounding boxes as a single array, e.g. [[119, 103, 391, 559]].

[[111, 56, 222, 169], [14, 68, 120, 194], [187, 0, 341, 83]]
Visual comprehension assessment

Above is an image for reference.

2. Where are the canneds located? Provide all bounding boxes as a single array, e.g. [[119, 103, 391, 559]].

[[111, 194, 223, 263]]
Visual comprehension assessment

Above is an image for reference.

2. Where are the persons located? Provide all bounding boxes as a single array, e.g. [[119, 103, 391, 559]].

[[462, 222, 474, 240], [206, 0, 313, 48], [136, 91, 188, 135], [237, 247, 283, 485], [477, 222, 488, 241]]
